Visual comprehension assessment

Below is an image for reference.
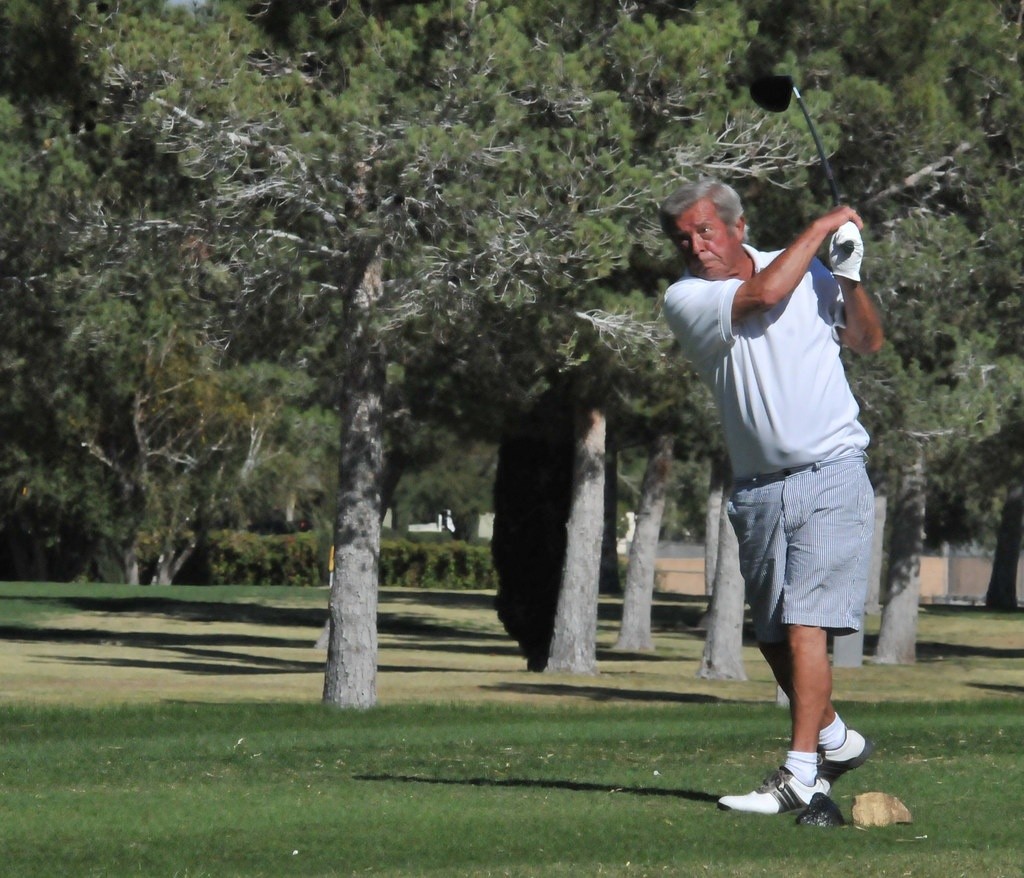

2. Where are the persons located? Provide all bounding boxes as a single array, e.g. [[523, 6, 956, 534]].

[[661, 178, 884, 818]]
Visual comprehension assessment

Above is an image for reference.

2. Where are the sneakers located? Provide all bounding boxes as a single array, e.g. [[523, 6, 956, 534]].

[[719, 766, 832, 815], [817, 727, 872, 783]]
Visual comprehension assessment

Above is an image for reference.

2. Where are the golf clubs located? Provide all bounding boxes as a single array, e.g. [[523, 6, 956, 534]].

[[747, 75, 856, 254]]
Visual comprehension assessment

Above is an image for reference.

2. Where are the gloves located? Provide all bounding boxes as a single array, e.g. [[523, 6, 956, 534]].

[[830, 222, 865, 283]]
